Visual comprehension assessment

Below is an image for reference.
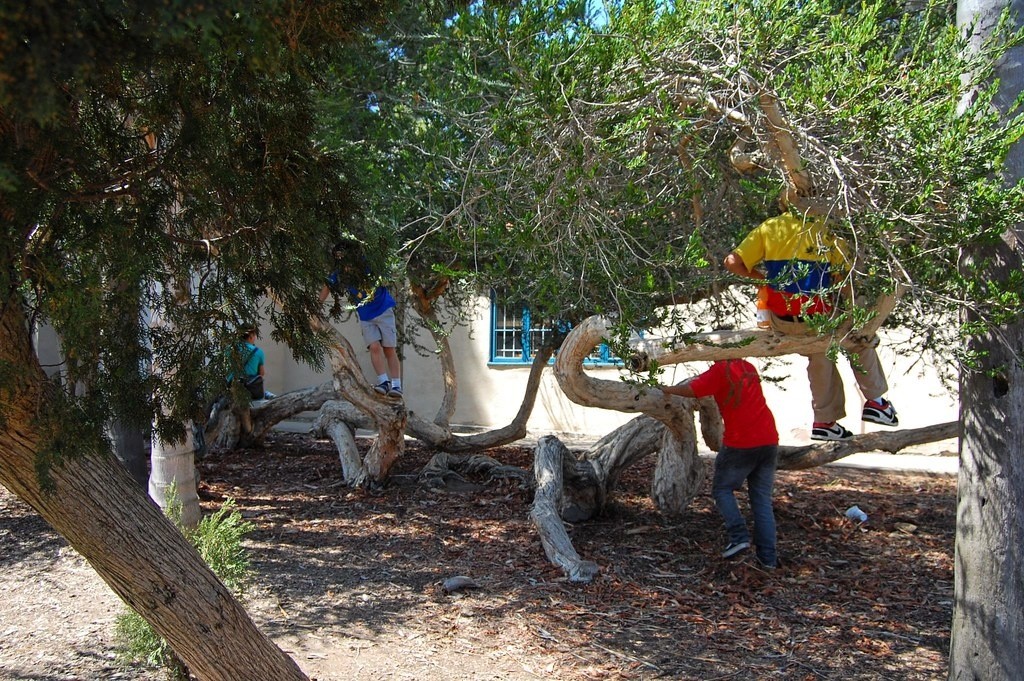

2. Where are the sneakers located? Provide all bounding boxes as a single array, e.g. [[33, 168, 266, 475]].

[[389, 385, 403, 398], [811, 421, 853, 441], [374, 381, 391, 395], [862, 397, 898, 426], [754, 553, 776, 571], [722, 540, 751, 559]]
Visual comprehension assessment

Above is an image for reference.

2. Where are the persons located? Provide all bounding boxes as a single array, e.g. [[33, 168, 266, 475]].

[[317, 243, 405, 399], [655, 326, 779, 571], [722, 179, 900, 441], [192, 323, 278, 432]]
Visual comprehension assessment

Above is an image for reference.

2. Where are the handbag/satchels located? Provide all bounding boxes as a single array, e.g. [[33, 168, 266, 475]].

[[238, 375, 264, 401]]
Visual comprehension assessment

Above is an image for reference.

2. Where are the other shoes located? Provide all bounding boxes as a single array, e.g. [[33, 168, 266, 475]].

[[265, 437, 276, 444]]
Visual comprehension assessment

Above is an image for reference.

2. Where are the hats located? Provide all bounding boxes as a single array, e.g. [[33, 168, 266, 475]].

[[332, 242, 353, 267]]
